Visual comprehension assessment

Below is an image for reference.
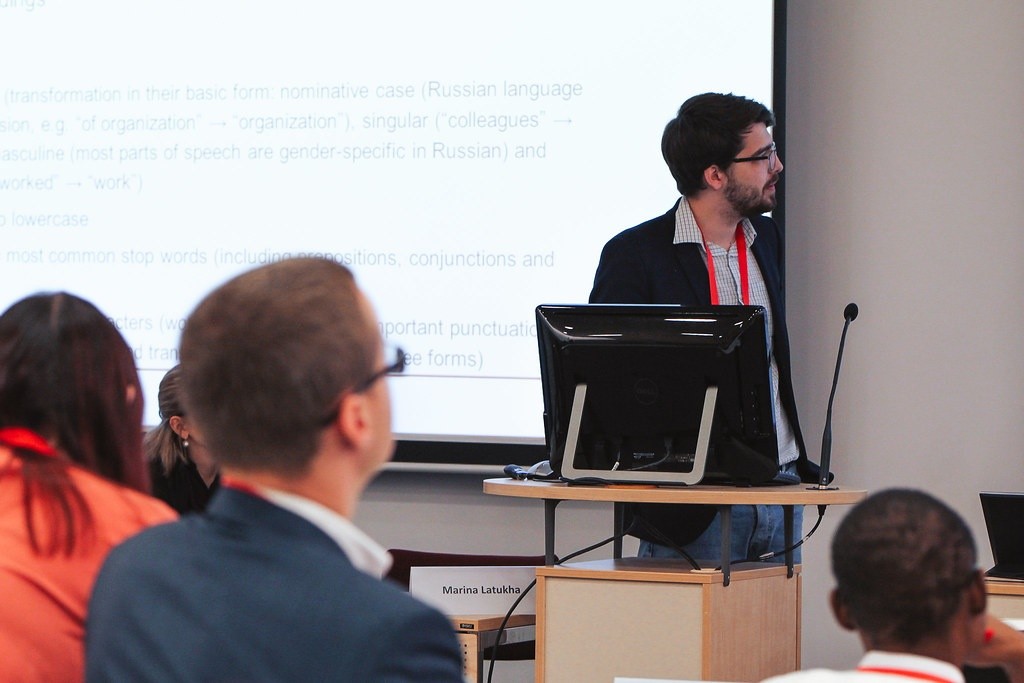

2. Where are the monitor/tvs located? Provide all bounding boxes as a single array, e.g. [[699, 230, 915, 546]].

[[534, 303, 780, 486]]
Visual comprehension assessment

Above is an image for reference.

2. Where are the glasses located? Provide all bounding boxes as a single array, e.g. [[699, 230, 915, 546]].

[[319, 346, 406, 428], [731, 142, 777, 170]]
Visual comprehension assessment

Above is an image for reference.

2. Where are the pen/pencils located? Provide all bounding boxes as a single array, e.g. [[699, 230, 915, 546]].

[[985, 628, 994, 642]]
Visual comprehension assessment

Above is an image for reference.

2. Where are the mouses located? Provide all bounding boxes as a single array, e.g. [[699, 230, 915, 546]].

[[529, 460, 557, 479]]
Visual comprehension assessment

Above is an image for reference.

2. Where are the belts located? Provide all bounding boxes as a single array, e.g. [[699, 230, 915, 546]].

[[779, 460, 794, 472]]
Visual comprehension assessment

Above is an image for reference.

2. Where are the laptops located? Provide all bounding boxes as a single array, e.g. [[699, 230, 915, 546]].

[[979, 491, 1024, 580]]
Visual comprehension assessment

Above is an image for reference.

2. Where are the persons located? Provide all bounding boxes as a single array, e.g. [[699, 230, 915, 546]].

[[0, 257, 470, 683], [588, 93, 834, 563], [762, 489, 1024, 683]]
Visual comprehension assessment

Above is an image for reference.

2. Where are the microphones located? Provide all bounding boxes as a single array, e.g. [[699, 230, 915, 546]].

[[818, 302, 859, 490]]
